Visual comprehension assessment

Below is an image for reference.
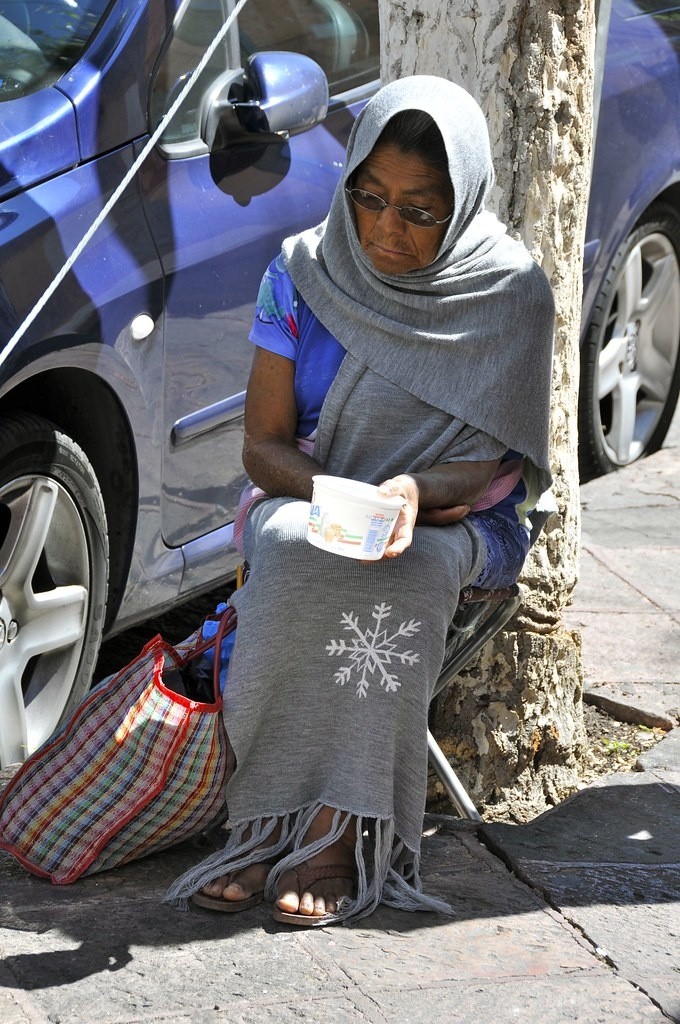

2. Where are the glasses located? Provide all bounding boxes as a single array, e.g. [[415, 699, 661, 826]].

[[345, 187, 453, 228]]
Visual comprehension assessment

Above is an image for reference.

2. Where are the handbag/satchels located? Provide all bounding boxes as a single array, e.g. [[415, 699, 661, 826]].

[[1, 604, 240, 884]]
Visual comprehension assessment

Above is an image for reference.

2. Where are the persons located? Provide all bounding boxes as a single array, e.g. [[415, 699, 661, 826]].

[[159, 75, 557, 932]]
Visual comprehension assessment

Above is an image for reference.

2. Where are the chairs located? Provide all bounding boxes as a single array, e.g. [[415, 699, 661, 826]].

[[232, 491, 559, 828], [234, 0, 370, 78]]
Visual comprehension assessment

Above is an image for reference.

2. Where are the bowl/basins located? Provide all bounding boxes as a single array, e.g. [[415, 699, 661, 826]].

[[307, 474, 407, 562]]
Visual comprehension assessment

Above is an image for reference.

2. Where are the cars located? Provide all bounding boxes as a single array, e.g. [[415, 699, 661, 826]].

[[0, 0, 680, 797]]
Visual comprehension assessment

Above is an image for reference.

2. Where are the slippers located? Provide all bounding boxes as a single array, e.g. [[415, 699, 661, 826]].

[[271, 860, 356, 925], [192, 853, 287, 911]]
